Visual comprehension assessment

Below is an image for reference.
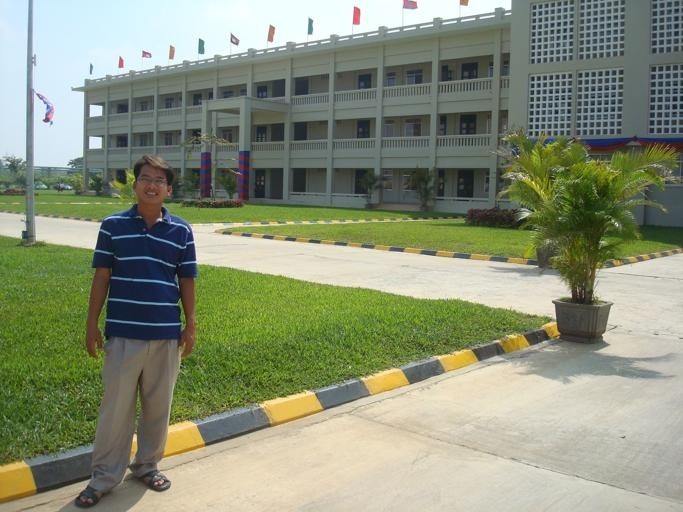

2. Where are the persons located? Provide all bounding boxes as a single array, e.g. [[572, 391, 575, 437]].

[[72, 154, 197, 508]]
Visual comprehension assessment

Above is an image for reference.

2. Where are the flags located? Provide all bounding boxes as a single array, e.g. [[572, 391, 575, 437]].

[[168, 46, 174, 59], [89, 64, 92, 74], [402, 0, 417, 9], [307, 17, 313, 35], [458, 0, 469, 6], [267, 25, 274, 42], [197, 38, 204, 54], [141, 51, 151, 58], [352, 7, 360, 25], [118, 57, 124, 68], [229, 33, 239, 46]]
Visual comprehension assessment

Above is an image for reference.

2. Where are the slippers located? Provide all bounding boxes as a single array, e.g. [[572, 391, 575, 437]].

[[75, 485, 103, 508], [139, 470, 170, 491]]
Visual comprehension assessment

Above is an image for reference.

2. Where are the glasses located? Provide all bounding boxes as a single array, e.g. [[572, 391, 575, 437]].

[[137, 177, 167, 185]]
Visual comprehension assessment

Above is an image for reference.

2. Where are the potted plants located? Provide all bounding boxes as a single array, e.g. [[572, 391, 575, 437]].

[[359, 170, 380, 209], [406, 162, 448, 212], [493, 126, 680, 346]]
